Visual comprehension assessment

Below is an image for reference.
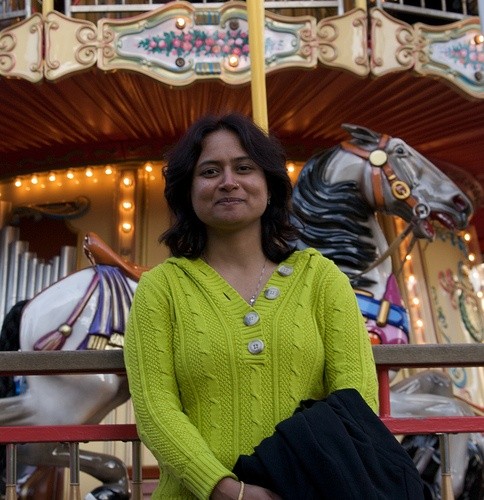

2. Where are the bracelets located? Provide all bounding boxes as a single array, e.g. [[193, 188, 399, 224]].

[[237, 480, 245, 500]]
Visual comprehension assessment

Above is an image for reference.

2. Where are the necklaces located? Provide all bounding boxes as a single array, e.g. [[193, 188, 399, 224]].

[[245, 255, 266, 306]]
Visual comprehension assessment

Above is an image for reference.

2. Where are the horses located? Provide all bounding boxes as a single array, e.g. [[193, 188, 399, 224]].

[[0, 121, 477, 499]]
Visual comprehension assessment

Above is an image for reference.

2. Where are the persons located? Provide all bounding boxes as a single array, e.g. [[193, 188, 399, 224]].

[[121, 114, 380, 500]]
